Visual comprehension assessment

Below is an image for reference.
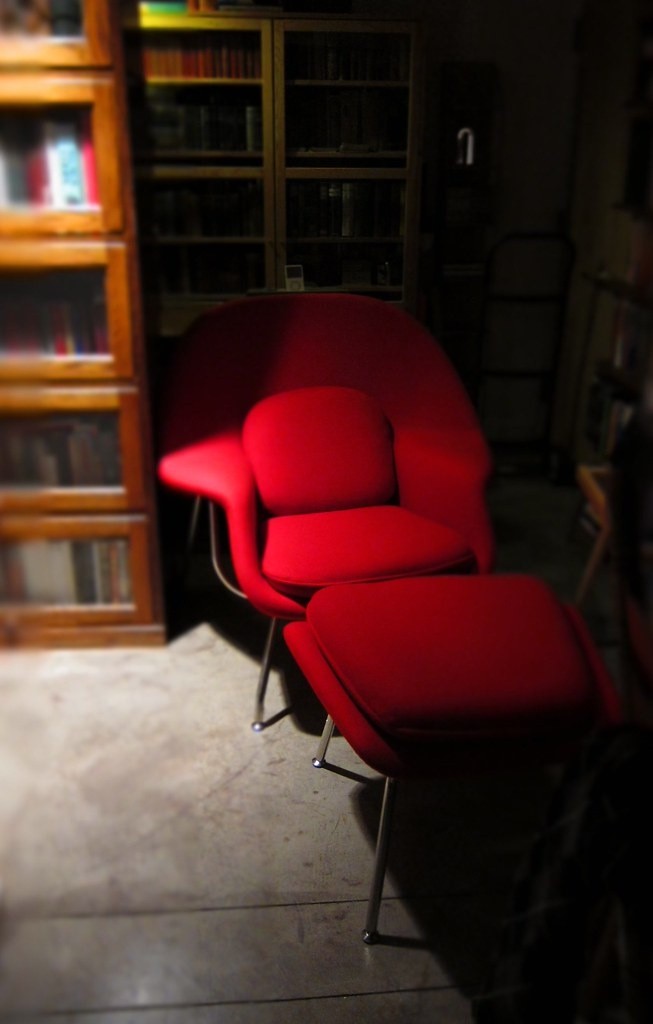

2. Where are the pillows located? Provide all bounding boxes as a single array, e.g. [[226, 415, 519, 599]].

[[244, 384, 399, 512]]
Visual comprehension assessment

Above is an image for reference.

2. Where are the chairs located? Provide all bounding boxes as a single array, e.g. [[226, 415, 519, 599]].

[[152, 286, 497, 731]]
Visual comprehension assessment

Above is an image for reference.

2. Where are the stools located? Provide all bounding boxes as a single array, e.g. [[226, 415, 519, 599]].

[[274, 577, 633, 984]]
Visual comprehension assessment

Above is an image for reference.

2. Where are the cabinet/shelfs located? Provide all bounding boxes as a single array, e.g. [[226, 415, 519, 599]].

[[0, 0, 166, 651], [134, 7, 421, 346]]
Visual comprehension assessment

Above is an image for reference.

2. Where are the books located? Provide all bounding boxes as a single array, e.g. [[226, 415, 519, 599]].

[[291, 246, 403, 286], [284, 87, 408, 145], [288, 181, 405, 236], [0, 414, 122, 488], [590, 233, 653, 460], [0, 108, 101, 207], [0, 296, 110, 357], [140, 184, 263, 237], [0, 538, 132, 606], [0, 0, 83, 41], [143, 43, 260, 80], [152, 245, 264, 293], [146, 105, 262, 153], [284, 34, 408, 80]]
